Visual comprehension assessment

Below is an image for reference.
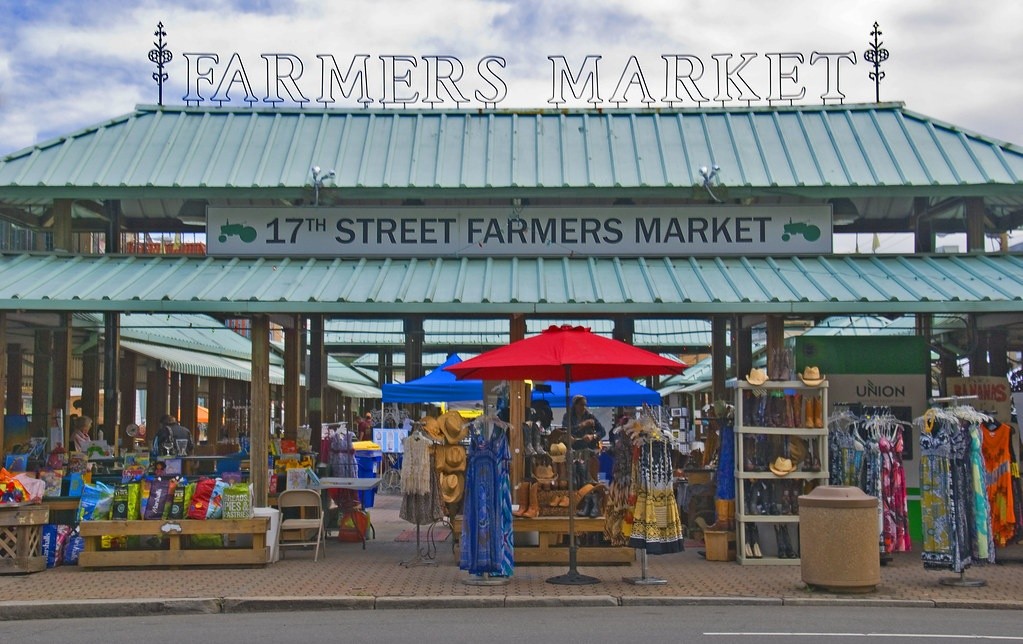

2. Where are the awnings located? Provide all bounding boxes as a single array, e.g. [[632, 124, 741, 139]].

[[101, 338, 306, 386], [327, 380, 383, 398]]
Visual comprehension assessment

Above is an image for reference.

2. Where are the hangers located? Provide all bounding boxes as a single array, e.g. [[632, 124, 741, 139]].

[[698, 397, 736, 434], [828, 394, 1017, 436], [610, 407, 682, 446], [399, 401, 514, 445], [279, 419, 358, 443]]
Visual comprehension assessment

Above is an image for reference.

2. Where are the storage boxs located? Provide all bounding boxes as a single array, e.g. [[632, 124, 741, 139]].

[[695, 516, 738, 563], [5, 439, 181, 498]]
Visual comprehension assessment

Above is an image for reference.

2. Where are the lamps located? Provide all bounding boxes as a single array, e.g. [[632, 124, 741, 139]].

[[176, 198, 212, 226], [826, 197, 861, 226], [38, 204, 56, 229]]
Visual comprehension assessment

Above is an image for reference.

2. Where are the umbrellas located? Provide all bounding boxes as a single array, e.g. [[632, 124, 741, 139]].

[[178, 405, 224, 423], [444, 325, 688, 583]]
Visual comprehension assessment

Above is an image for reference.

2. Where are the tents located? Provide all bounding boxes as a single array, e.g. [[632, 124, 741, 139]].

[[380, 354, 484, 491], [532, 377, 661, 431]]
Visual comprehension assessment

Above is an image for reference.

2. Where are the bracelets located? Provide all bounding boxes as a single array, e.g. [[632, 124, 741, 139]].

[[594, 434, 597, 439]]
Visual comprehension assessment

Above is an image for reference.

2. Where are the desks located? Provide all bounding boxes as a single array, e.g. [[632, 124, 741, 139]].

[[306, 477, 384, 550], [176, 453, 233, 474]]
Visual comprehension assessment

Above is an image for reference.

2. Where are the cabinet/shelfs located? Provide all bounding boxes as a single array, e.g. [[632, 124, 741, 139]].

[[734, 378, 830, 567]]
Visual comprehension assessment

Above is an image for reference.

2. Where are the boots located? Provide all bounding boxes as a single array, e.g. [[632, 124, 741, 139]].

[[801, 439, 820, 471], [523, 482, 540, 517], [744, 394, 823, 427], [590, 494, 599, 516], [512, 483, 530, 516], [577, 496, 592, 515], [773, 523, 796, 558], [742, 437, 766, 471], [748, 479, 799, 515], [743, 526, 760, 558]]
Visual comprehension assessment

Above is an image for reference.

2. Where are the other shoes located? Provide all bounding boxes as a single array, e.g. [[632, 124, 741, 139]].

[[548, 494, 560, 506], [707, 519, 737, 531], [559, 496, 569, 507]]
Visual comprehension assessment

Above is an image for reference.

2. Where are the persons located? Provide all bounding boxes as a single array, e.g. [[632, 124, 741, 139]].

[[563, 395, 607, 482], [70, 416, 93, 449], [356, 413, 373, 440], [152, 415, 194, 465]]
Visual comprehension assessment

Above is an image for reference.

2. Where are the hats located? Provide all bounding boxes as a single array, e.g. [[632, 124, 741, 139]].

[[798, 367, 826, 387], [768, 458, 796, 476], [418, 416, 444, 442], [443, 446, 466, 473], [746, 368, 769, 386], [438, 473, 464, 503], [437, 411, 467, 445]]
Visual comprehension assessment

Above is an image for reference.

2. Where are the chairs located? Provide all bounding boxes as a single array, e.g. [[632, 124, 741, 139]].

[[272, 489, 326, 564]]
